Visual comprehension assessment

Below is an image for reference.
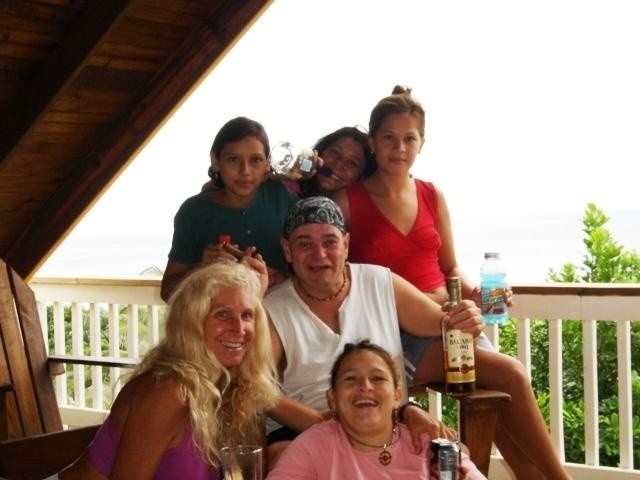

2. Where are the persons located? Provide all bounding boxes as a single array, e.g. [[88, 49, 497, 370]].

[[263, 339, 487, 480], [262, 196, 485, 480], [160, 116, 301, 302], [37, 261, 456, 480], [333, 85, 574, 480], [278, 125, 377, 200]]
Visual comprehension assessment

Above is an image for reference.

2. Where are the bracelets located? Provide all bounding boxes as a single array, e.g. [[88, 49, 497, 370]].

[[398, 400, 424, 421]]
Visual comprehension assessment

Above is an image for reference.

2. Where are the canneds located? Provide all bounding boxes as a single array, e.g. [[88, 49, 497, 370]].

[[429, 437, 462, 480]]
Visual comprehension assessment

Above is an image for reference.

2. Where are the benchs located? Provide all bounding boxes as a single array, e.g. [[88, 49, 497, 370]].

[[1, 383, 512, 480]]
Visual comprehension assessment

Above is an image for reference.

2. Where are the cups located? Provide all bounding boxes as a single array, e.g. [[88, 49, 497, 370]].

[[219, 444, 264, 480]]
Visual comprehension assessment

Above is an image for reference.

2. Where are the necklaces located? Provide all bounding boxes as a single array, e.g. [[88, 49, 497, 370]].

[[296, 268, 346, 302], [344, 423, 401, 466]]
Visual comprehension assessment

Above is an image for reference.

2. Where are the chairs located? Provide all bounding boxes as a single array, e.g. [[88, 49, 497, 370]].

[[0, 258, 142, 480]]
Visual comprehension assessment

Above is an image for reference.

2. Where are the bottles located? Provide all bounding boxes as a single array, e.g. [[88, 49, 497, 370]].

[[479, 251, 510, 326], [441, 276, 477, 398], [268, 139, 333, 182]]
